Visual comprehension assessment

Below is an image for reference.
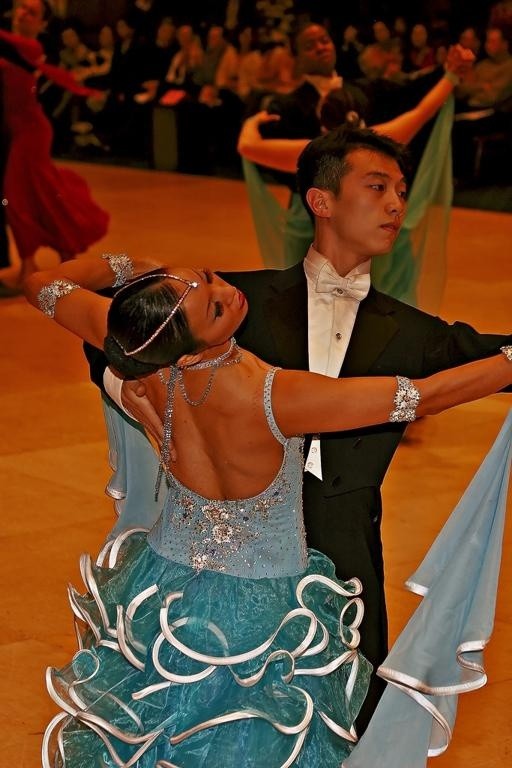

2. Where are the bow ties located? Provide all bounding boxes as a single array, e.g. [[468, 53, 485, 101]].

[[314, 264, 372, 302]]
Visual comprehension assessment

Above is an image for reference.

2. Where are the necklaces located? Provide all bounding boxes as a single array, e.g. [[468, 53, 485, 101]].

[[174, 338, 244, 409]]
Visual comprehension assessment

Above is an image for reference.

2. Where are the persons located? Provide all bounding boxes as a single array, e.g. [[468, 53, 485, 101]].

[[9, 251, 512, 768], [1, 1, 512, 216], [0, 0, 105, 297], [237, 43, 477, 450], [2, 0, 43, 298], [78, 124, 510, 744]]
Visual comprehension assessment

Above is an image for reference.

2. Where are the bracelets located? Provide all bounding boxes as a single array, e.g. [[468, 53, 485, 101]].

[[98, 250, 136, 291], [497, 344, 510, 357]]
